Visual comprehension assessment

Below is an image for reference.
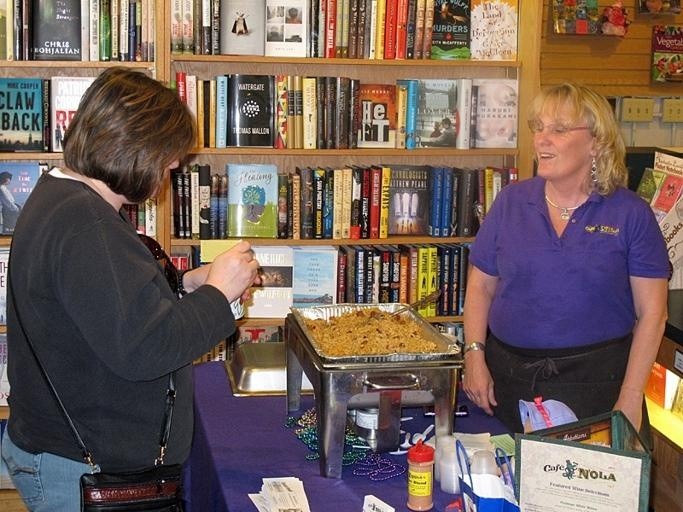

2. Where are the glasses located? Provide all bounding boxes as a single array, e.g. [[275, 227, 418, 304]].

[[137, 234, 180, 292], [528, 118, 589, 136]]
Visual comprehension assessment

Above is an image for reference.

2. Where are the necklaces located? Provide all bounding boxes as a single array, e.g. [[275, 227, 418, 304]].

[[544, 196, 589, 223], [286, 406, 406, 483]]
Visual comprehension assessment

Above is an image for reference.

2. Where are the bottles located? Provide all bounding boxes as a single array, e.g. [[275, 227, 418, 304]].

[[472, 450, 501, 480], [406, 440, 434, 510], [441, 448, 463, 494], [435, 437, 454, 481]]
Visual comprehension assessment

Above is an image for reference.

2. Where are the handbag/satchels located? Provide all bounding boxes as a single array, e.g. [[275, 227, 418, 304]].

[[80, 464, 186, 512]]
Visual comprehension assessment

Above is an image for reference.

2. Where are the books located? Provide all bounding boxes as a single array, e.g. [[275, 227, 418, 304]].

[[171, 166, 518, 238], [171, 71, 516, 149], [361, 493, 395, 512], [632, 149, 682, 450], [172, 240, 474, 361], [552, 0, 602, 36], [637, 1, 682, 16], [650, 22, 683, 83], [0, 1, 157, 400], [171, 2, 517, 59]]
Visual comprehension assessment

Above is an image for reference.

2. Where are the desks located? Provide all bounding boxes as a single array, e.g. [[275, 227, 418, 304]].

[[185, 361, 519, 512]]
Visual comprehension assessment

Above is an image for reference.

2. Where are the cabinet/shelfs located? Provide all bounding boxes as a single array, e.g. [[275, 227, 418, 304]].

[[0, 0, 544, 420]]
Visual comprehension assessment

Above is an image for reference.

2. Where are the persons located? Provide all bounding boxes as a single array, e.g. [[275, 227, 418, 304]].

[[1, 62, 266, 511], [458, 78, 675, 434]]
